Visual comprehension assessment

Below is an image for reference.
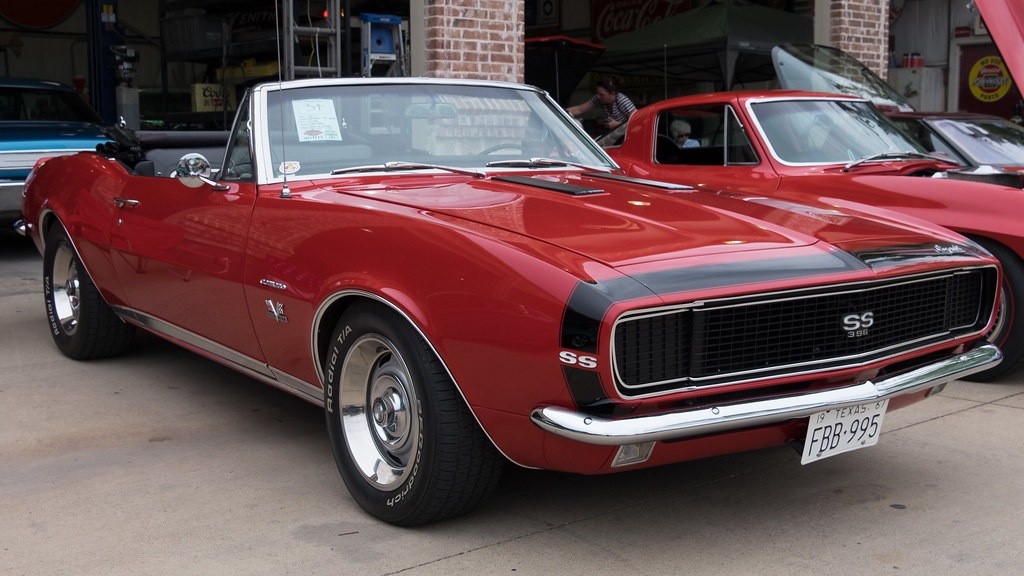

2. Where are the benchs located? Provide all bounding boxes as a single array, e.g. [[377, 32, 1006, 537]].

[[130, 131, 383, 182]]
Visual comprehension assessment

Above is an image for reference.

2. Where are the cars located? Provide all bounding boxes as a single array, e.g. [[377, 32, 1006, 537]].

[[600, 90, 1024, 383], [0, 77, 116, 224], [823, 112, 1024, 167]]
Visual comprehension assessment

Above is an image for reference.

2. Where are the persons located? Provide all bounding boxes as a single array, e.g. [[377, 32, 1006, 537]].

[[671, 121, 700, 149], [566, 76, 637, 145]]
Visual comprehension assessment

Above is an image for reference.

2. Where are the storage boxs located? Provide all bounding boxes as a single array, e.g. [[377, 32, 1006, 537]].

[[216, 54, 283, 82], [190, 83, 237, 113], [161, 12, 223, 52]]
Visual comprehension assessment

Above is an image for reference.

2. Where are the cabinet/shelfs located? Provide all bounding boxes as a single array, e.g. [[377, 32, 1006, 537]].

[[161, 40, 283, 131]]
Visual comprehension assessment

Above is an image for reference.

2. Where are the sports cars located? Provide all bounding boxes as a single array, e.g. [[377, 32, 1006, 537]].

[[14, 78, 1007, 529]]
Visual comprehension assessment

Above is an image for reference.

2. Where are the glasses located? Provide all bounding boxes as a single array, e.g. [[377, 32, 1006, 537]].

[[674, 133, 690, 138]]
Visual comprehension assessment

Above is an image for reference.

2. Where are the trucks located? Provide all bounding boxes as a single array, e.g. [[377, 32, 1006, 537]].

[[599, 35, 916, 152]]
